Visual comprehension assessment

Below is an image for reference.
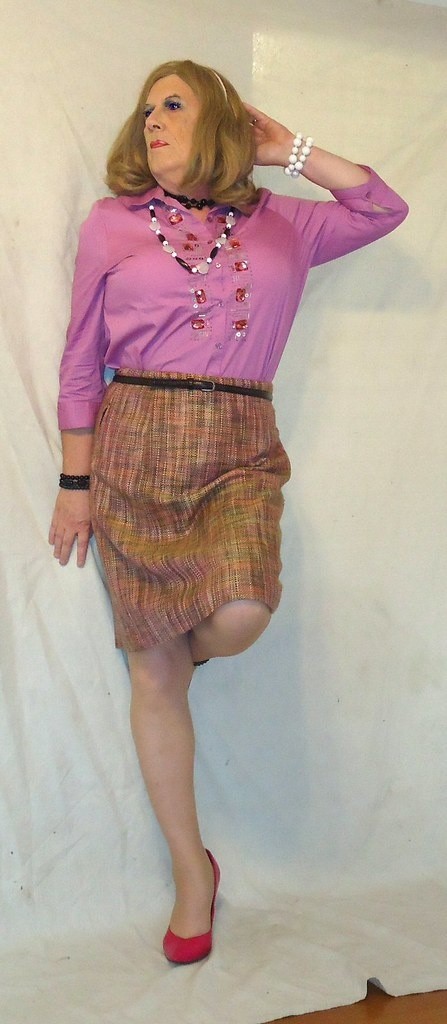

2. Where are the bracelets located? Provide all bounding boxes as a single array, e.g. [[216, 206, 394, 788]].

[[285, 132, 313, 178], [59, 474, 90, 490]]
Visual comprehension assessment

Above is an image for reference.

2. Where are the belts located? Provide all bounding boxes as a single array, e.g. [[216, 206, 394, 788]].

[[112, 374, 273, 402]]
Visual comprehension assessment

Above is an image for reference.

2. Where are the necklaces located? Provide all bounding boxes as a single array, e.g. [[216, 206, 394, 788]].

[[148, 200, 233, 274], [163, 190, 217, 210]]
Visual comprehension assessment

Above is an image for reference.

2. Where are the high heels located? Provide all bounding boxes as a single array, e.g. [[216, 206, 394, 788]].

[[163, 849, 220, 964]]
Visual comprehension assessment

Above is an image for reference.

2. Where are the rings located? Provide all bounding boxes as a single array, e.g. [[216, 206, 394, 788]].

[[252, 119, 256, 124]]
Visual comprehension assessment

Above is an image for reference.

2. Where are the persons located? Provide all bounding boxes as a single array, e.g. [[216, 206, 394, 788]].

[[47, 58, 409, 964]]
